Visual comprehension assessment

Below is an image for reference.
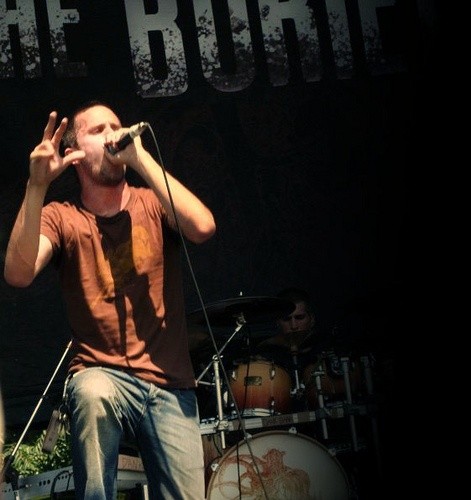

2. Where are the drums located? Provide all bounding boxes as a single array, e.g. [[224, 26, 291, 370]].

[[303, 360, 355, 402], [207, 430, 351, 500], [227, 360, 292, 418]]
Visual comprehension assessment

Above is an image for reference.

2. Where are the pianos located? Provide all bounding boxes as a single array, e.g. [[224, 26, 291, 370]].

[[2, 452, 152, 500]]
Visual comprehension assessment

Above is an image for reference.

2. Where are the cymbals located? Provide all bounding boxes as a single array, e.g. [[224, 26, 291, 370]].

[[187, 293, 280, 327], [265, 327, 323, 350]]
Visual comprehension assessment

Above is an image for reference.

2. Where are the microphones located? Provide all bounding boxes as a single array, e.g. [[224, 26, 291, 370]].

[[108, 122, 146, 155]]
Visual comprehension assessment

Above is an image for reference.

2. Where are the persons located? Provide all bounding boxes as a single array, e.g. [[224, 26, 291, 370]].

[[1, 101, 219, 500], [269, 292, 322, 344]]
